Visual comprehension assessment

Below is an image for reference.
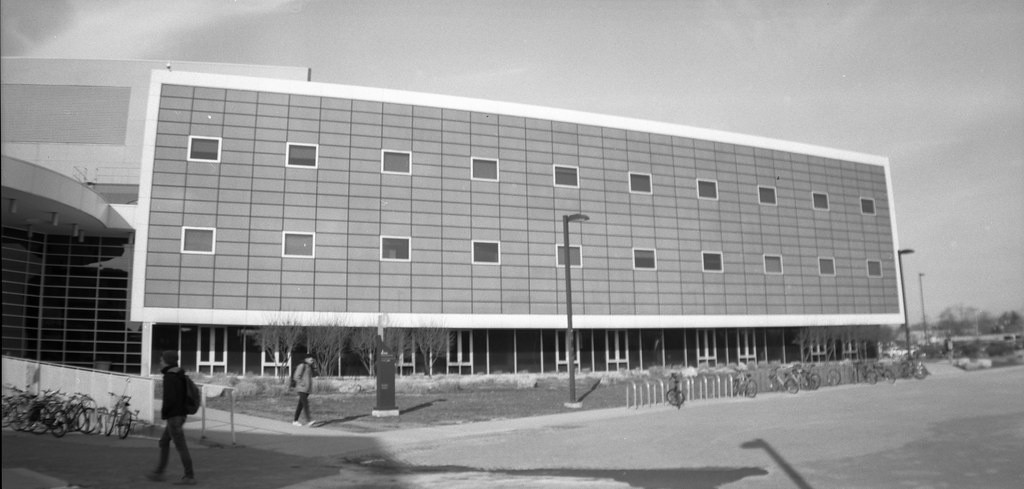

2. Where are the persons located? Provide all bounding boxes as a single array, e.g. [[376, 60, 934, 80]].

[[945, 335, 953, 364], [146, 350, 200, 486], [292, 354, 316, 427]]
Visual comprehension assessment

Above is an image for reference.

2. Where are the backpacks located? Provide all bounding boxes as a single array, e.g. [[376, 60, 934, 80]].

[[166, 373, 202, 415], [290, 362, 306, 386]]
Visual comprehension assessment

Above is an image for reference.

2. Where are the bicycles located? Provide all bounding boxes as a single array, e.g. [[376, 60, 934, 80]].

[[104, 391, 133, 438], [769, 364, 821, 393], [732, 366, 758, 400], [2, 385, 99, 437], [899, 356, 929, 380], [665, 370, 685, 410], [853, 360, 897, 385]]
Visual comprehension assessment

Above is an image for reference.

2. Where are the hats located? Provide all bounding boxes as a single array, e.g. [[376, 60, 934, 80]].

[[162, 350, 179, 365]]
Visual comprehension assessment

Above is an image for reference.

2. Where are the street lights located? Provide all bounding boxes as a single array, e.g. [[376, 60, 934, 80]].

[[562, 213, 590, 408], [918, 272, 929, 346], [898, 248, 916, 372]]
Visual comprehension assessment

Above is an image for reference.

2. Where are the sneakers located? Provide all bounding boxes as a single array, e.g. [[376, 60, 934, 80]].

[[292, 421, 302, 427], [173, 474, 196, 485], [146, 471, 165, 480], [308, 420, 316, 426]]
